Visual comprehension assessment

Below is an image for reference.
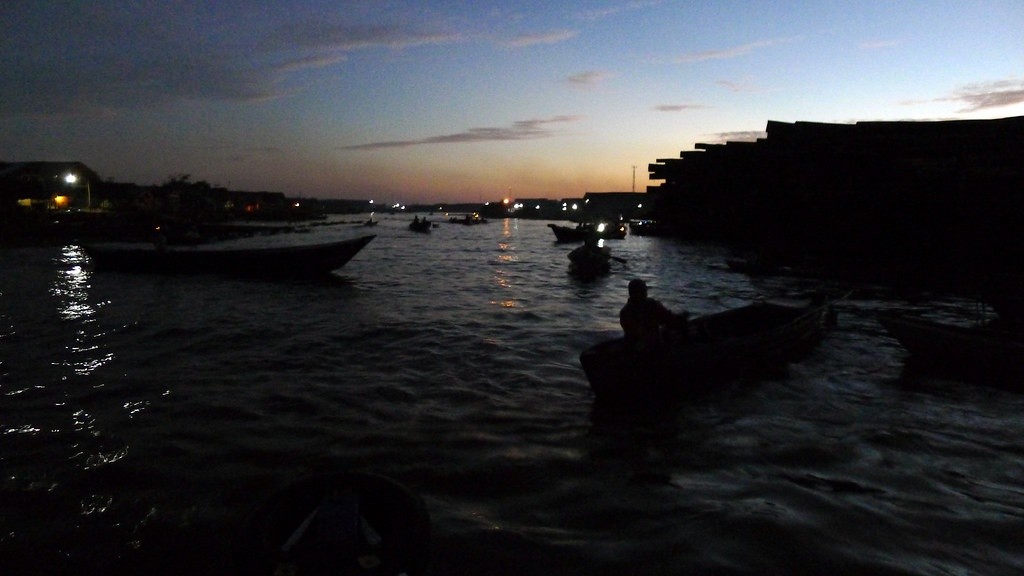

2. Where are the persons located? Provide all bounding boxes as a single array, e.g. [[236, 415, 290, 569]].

[[561, 212, 652, 267], [414, 215, 419, 225], [422, 217, 428, 223], [619, 280, 688, 343], [449, 214, 483, 225]]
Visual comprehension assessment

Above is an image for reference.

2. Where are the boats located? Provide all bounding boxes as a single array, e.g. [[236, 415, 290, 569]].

[[876, 306, 1023, 394], [69, 231, 379, 284], [410, 220, 431, 232], [567, 240, 610, 263], [548, 222, 627, 244], [577, 301, 822, 405]]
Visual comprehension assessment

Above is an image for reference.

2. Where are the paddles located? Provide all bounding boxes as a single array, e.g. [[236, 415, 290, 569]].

[[609, 255, 627, 264]]
